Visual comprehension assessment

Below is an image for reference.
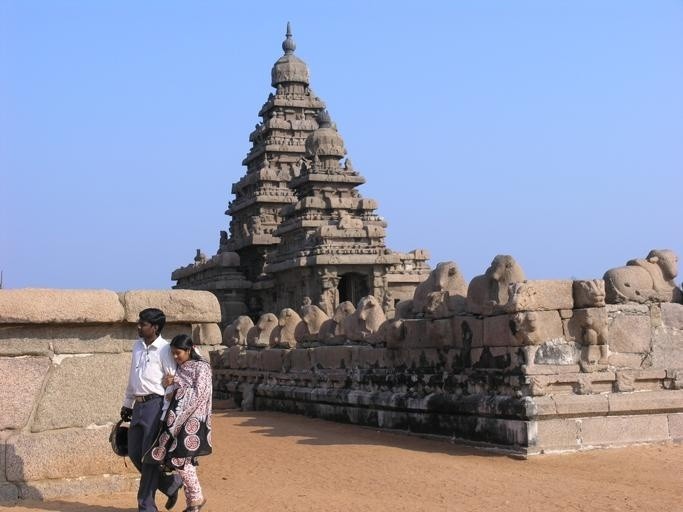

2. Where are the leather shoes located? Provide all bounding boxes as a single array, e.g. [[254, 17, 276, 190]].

[[165, 482, 183, 509]]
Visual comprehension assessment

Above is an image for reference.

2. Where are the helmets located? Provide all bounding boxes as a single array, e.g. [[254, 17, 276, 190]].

[[111, 427, 128, 456]]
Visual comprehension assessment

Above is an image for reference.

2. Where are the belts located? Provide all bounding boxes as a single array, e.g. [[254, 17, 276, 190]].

[[135, 394, 159, 403]]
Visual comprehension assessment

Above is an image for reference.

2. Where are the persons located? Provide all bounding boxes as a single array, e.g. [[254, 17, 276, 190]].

[[161, 334, 213, 512], [120, 308, 179, 512]]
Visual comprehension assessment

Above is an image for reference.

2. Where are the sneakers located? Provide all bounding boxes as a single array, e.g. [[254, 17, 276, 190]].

[[185, 500, 206, 512]]
